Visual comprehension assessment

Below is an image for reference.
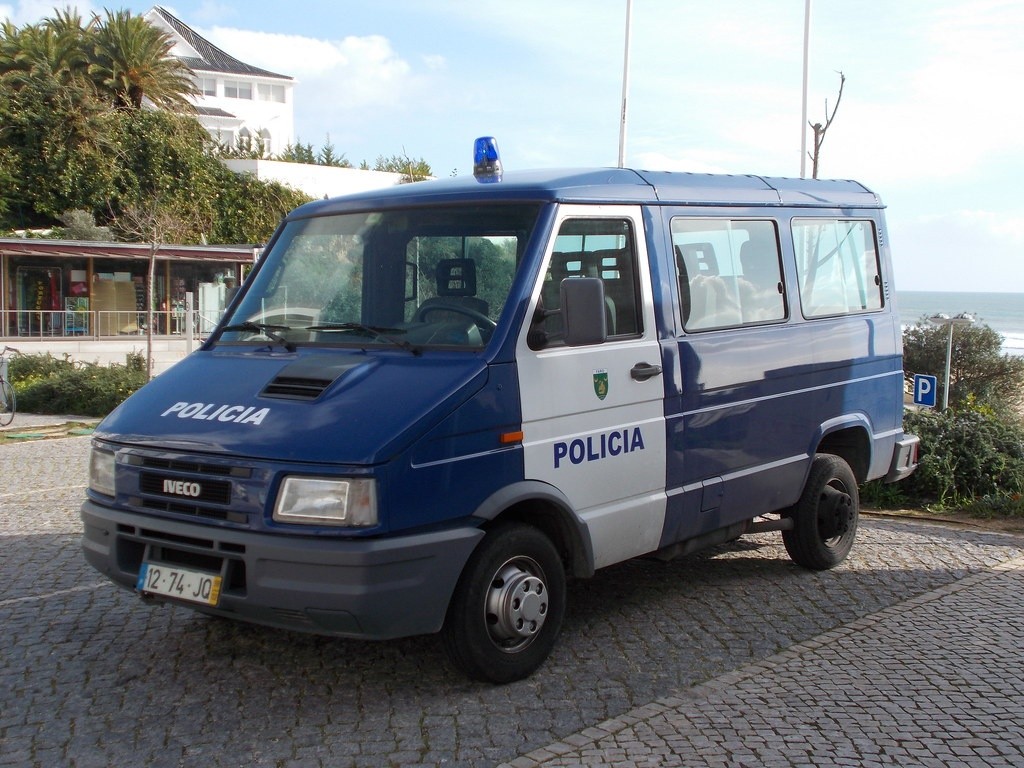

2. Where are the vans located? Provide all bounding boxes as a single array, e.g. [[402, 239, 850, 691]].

[[77, 134, 919, 684]]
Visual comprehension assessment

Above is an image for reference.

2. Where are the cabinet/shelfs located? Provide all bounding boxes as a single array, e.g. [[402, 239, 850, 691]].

[[64, 297, 89, 335]]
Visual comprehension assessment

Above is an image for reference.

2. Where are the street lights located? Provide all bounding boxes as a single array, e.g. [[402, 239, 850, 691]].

[[930, 314, 976, 416]]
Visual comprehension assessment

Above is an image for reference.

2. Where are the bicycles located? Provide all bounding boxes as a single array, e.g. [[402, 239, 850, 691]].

[[0, 346, 22, 429]]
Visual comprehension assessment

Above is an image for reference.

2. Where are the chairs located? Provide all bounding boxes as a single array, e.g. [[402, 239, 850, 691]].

[[413, 259, 488, 328], [678, 243, 719, 278], [540, 249, 637, 335], [740, 240, 779, 275]]
